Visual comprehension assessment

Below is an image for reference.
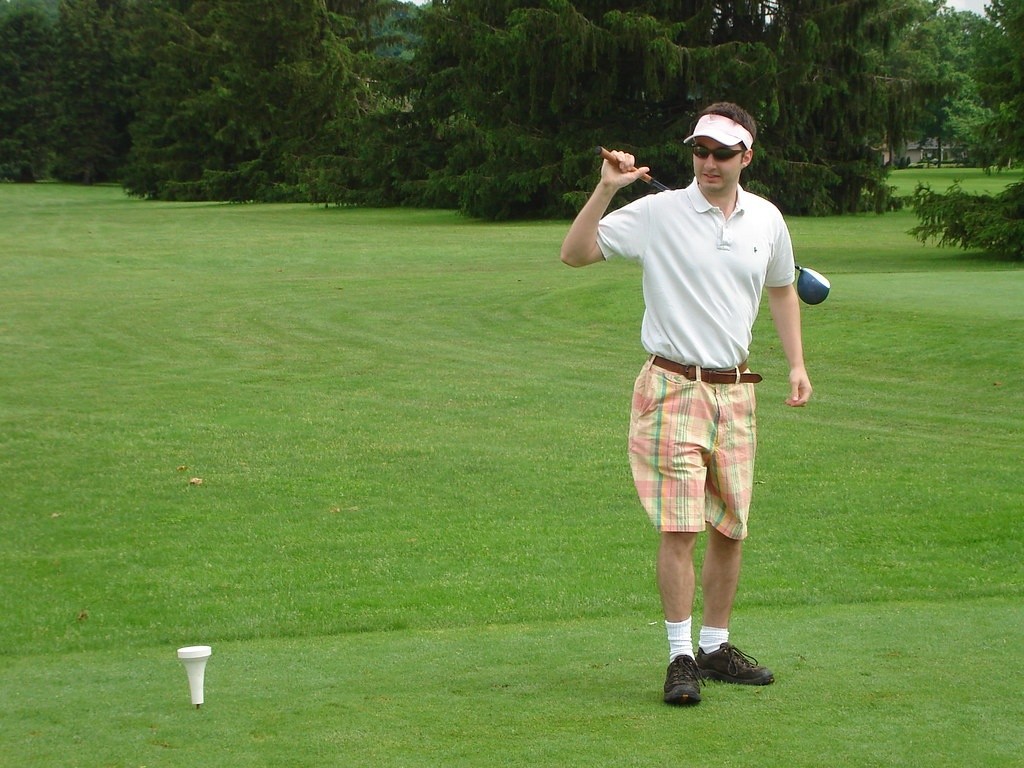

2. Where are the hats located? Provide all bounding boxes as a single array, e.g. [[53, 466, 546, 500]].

[[683, 114, 754, 151]]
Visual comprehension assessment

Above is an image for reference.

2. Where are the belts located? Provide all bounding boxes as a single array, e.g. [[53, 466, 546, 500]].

[[649, 354, 763, 384]]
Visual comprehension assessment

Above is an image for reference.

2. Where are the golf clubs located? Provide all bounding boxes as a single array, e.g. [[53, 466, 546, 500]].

[[590, 143, 831, 306]]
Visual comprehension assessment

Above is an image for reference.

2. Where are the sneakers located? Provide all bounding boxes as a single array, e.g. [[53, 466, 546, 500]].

[[695, 642, 774, 685], [664, 655, 706, 703]]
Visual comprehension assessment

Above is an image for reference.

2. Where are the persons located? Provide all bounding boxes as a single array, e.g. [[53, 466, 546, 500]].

[[560, 103, 812, 703]]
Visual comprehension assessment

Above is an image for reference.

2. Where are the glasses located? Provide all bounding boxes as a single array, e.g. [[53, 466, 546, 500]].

[[691, 143, 745, 160]]
